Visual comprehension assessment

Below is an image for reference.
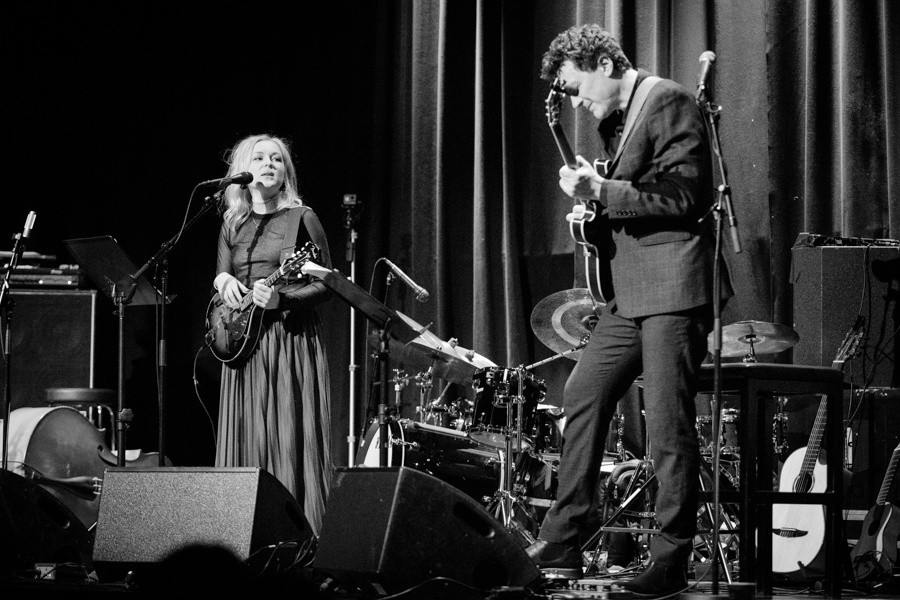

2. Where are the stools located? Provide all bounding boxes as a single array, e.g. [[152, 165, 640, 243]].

[[688, 359, 842, 598], [45, 388, 115, 454]]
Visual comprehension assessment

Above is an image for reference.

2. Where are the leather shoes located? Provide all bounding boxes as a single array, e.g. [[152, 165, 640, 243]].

[[625, 561, 690, 600], [525, 540, 584, 579]]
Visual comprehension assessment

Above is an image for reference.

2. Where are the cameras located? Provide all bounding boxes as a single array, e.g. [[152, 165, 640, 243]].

[[344, 194, 357, 206]]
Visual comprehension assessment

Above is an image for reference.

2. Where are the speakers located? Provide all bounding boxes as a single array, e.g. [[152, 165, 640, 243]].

[[0, 288, 96, 425], [0, 465, 548, 591]]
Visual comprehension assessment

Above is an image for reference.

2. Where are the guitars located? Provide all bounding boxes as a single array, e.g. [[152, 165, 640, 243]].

[[544, 74, 618, 303], [754, 312, 871, 581], [850, 441, 900, 583], [204, 240, 323, 370]]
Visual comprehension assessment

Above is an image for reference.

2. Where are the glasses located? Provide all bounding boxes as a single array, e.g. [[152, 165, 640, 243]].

[[564, 81, 580, 97]]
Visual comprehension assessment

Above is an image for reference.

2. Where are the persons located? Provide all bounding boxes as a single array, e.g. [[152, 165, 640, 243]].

[[523, 25, 735, 598], [132, 545, 334, 600], [214, 135, 334, 541]]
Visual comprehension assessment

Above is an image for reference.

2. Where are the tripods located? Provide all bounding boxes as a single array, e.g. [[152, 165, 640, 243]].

[[484, 333, 741, 583]]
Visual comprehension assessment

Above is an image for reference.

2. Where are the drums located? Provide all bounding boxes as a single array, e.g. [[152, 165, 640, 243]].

[[467, 365, 548, 453], [529, 403, 625, 466], [355, 414, 517, 517], [696, 413, 786, 462]]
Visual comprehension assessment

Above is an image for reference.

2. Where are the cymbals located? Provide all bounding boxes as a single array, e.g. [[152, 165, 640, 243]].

[[395, 308, 468, 363], [707, 317, 801, 359], [456, 346, 499, 369], [529, 286, 606, 361]]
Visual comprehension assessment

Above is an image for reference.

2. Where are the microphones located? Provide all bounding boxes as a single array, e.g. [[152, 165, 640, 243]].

[[15, 211, 38, 265], [199, 171, 253, 188], [694, 51, 716, 100], [384, 259, 429, 303]]
